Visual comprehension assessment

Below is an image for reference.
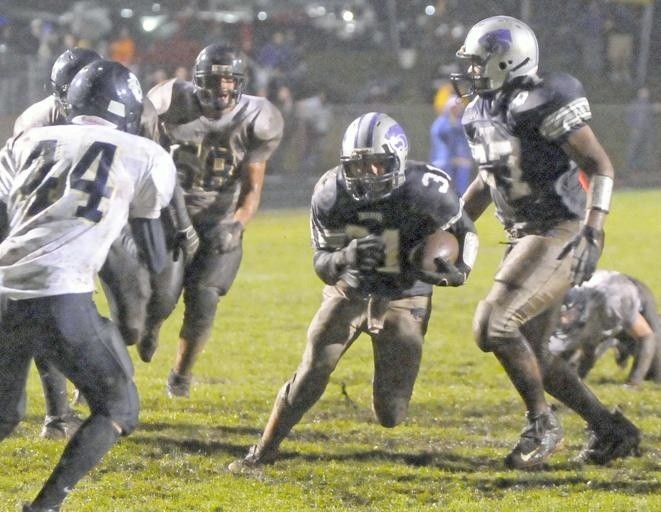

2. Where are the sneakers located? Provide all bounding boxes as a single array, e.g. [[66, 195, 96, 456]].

[[574, 411, 640, 467], [507, 409, 561, 468], [41, 407, 89, 440], [168, 370, 191, 398], [226, 447, 273, 475]]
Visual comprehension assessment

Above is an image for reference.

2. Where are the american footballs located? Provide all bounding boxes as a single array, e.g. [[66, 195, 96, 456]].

[[409, 229, 457, 274]]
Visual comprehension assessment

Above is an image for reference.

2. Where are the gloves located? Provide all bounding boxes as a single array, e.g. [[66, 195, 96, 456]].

[[419, 257, 471, 288], [209, 220, 243, 254], [173, 225, 200, 265], [558, 221, 604, 287], [336, 236, 386, 276]]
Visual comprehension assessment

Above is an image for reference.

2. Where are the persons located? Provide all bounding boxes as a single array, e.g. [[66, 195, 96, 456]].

[[11, 45, 201, 446], [137, 40, 288, 401], [549, 265, 661, 390], [460, 14, 644, 470], [0, 58, 177, 512], [0, 0, 660, 203], [225, 110, 484, 478]]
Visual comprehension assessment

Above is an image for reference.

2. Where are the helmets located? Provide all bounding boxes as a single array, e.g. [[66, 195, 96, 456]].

[[50, 49, 101, 97], [344, 112, 407, 201], [62, 58, 144, 135], [556, 292, 589, 338], [450, 15, 541, 93], [193, 43, 247, 103]]
